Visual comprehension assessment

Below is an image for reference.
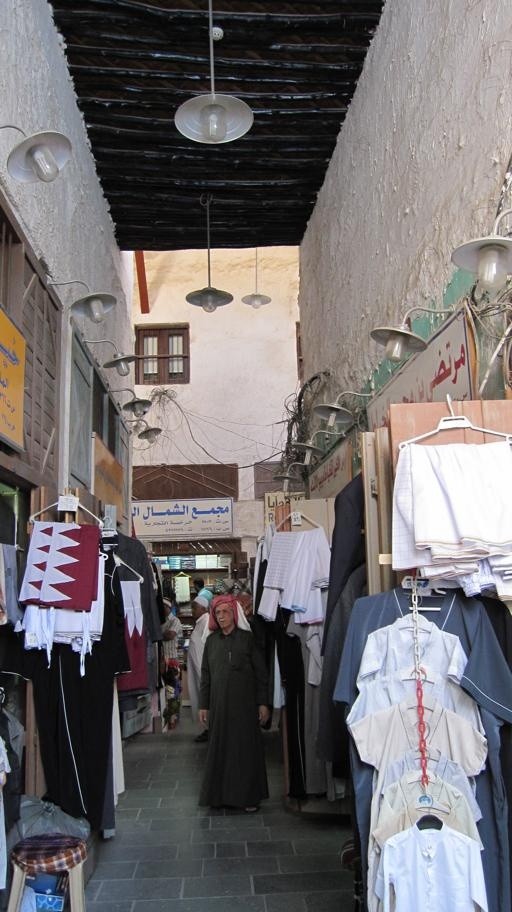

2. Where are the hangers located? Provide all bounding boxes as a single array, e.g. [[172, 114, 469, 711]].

[[385, 573, 471, 834], [98, 549, 108, 561], [114, 555, 145, 585], [102, 514, 119, 538], [395, 395, 512, 449], [272, 498, 321, 535], [172, 563, 192, 579], [30, 497, 104, 529]]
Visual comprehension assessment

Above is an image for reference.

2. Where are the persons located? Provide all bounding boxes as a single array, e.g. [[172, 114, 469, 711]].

[[161, 592, 185, 727], [186, 576, 267, 743], [197, 594, 274, 819]]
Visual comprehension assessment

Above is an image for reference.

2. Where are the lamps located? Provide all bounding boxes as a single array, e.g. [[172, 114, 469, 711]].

[[80, 338, 137, 377], [369, 306, 457, 361], [273, 462, 322, 492], [171, 0, 256, 150], [186, 192, 234, 313], [108, 385, 152, 418], [241, 200, 272, 311], [1, 120, 76, 186], [448, 208, 510, 291], [124, 419, 162, 443], [291, 430, 347, 465], [309, 389, 372, 427], [43, 273, 118, 326]]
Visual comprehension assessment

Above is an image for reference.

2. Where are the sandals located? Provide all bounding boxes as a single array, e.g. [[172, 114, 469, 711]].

[[194, 729, 208, 742]]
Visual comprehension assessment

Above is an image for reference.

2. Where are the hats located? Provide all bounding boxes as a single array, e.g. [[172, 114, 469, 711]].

[[193, 595, 209, 609], [163, 597, 173, 608]]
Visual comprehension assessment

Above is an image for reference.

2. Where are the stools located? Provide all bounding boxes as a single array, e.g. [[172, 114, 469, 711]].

[[5, 832, 89, 912]]
